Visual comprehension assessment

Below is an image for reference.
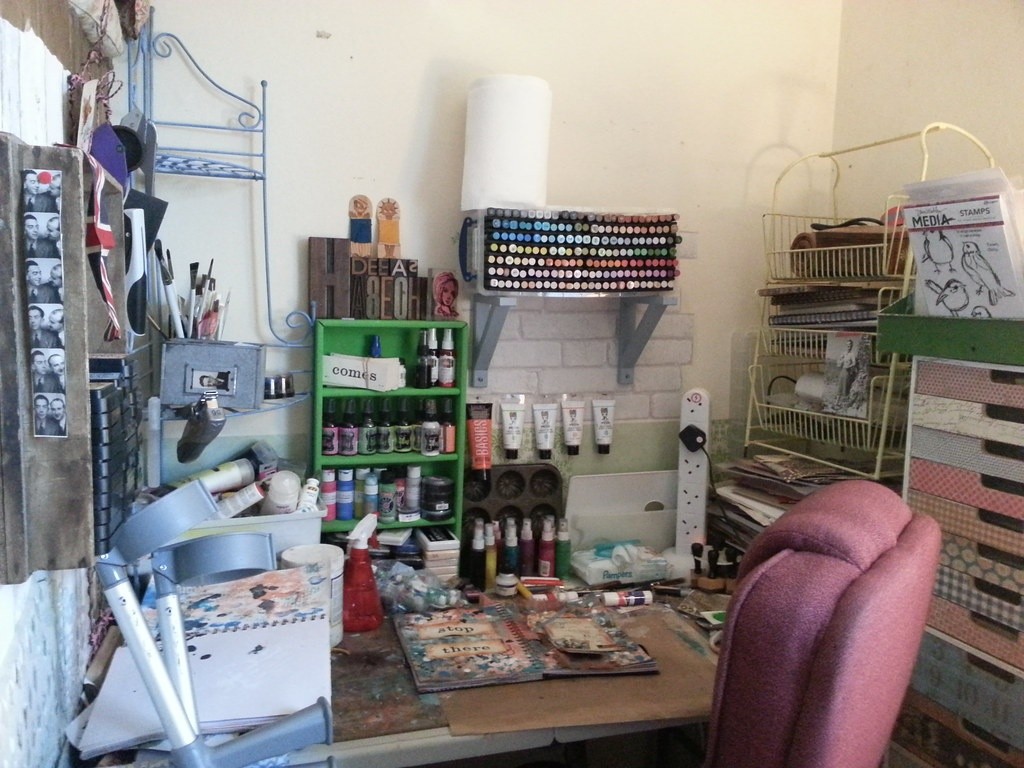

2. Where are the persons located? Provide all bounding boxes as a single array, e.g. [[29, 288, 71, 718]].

[[199, 372, 229, 389], [21, 170, 68, 436]]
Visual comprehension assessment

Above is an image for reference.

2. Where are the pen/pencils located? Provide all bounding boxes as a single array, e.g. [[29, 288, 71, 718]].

[[196, 258, 214, 325], [146, 314, 170, 341]]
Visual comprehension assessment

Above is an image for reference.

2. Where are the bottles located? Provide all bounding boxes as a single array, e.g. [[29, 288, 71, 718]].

[[358, 399, 377, 453], [339, 399, 358, 455], [377, 399, 394, 453], [412, 400, 425, 452], [322, 398, 339, 455], [421, 400, 439, 456], [394, 399, 412, 452], [439, 398, 456, 453]]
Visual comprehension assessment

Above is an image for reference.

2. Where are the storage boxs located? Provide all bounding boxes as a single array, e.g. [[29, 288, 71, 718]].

[[331, 526, 461, 585], [126, 496, 330, 578], [89, 356, 144, 555]]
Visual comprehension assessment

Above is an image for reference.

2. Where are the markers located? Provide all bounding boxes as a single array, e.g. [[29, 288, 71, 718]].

[[521, 576, 563, 587], [517, 581, 532, 600]]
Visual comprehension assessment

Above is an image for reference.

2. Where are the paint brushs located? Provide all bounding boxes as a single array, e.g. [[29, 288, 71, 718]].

[[187, 262, 199, 339], [167, 249, 175, 339], [154, 239, 185, 339], [193, 274, 208, 318]]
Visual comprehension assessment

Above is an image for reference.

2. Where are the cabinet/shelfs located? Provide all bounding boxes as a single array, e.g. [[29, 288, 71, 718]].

[[0, 131, 161, 589], [743, 121, 996, 480], [310, 318, 469, 540], [125, 5, 312, 484]]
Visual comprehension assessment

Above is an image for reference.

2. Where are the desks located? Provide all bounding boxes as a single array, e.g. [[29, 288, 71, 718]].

[[96, 577, 719, 768]]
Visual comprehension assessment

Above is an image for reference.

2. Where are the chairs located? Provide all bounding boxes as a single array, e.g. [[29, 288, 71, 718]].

[[704, 479, 942, 768]]
